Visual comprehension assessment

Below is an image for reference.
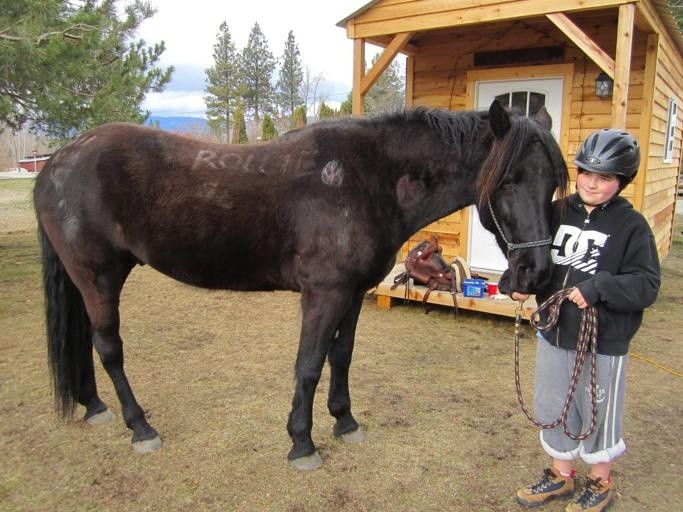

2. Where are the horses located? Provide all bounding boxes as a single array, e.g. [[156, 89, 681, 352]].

[[33, 97, 570, 472]]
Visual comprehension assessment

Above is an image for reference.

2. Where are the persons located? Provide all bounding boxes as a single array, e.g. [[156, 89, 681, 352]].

[[499, 131, 661, 512]]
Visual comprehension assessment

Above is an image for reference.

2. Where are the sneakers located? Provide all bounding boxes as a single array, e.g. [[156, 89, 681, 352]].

[[565, 475, 615, 512], [515, 466, 574, 507]]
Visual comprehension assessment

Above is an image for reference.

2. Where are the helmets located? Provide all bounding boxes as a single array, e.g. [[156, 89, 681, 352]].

[[574, 128, 640, 181]]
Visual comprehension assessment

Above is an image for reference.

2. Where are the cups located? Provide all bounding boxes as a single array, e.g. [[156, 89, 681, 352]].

[[487, 282, 498, 297]]
